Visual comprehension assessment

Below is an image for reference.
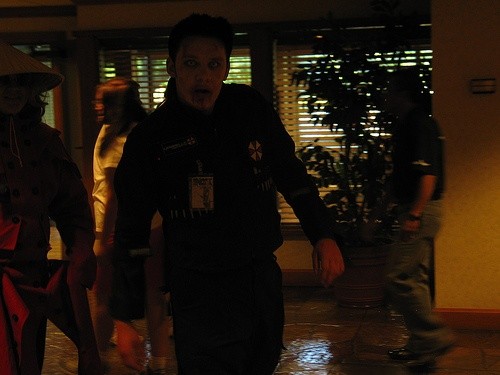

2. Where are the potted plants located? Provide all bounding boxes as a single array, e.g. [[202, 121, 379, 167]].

[[289, 9, 432, 309]]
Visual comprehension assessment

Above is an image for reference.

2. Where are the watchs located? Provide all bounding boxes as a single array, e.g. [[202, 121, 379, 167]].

[[405, 213, 421, 221]]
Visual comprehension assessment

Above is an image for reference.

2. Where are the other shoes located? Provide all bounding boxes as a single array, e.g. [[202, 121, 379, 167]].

[[144, 359, 167, 375], [98, 361, 110, 375], [100, 342, 116, 352]]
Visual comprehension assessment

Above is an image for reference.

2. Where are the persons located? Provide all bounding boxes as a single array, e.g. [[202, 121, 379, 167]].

[[90, 78, 167, 375], [0, 37, 97, 375], [363, 70, 458, 366], [107, 12, 345, 375]]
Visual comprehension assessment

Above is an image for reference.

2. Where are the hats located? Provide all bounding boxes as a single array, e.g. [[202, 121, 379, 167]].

[[0, 39, 64, 93]]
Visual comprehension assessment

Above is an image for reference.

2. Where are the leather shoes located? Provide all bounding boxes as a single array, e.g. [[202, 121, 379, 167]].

[[388, 343, 450, 362]]
